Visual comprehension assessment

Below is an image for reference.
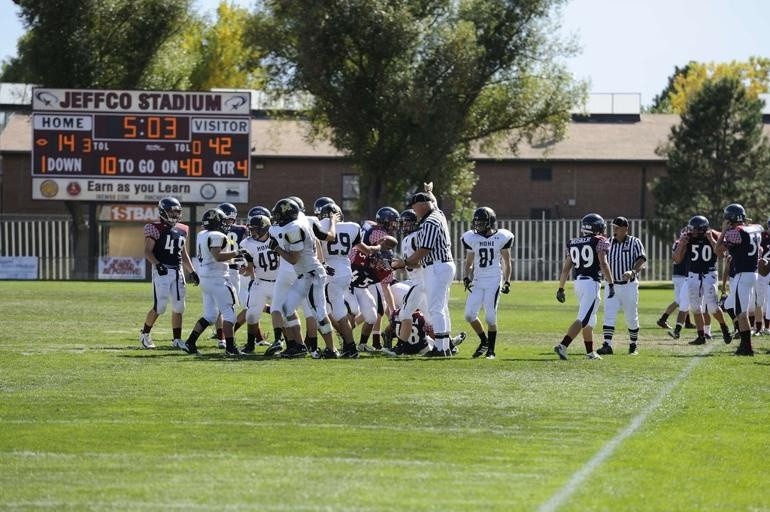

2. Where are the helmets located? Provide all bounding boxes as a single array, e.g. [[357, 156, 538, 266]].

[[400, 208, 418, 235], [687, 215, 710, 238], [723, 204, 747, 224], [376, 206, 402, 236], [159, 197, 343, 240], [581, 214, 606, 238], [472, 207, 498, 237]]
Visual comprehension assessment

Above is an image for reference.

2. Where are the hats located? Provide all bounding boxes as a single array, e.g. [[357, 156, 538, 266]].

[[609, 216, 630, 229], [406, 193, 432, 208]]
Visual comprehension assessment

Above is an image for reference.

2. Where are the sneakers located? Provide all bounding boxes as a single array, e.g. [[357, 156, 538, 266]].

[[629, 343, 640, 355], [172, 333, 466, 359], [554, 345, 569, 359], [596, 342, 615, 355], [484, 352, 496, 359], [472, 339, 488, 359], [585, 351, 604, 360], [656, 319, 770, 355]]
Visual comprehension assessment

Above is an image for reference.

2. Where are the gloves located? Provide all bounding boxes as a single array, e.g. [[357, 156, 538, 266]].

[[138, 330, 156, 349], [267, 236, 280, 251], [320, 261, 336, 277], [556, 287, 566, 304], [155, 262, 169, 277], [190, 271, 200, 286], [607, 283, 616, 298], [235, 249, 252, 262], [464, 277, 473, 293], [500, 282, 511, 294]]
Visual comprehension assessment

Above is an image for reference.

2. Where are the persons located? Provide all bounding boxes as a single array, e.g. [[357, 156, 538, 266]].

[[183, 181, 466, 359], [460, 206, 515, 358], [657, 203, 770, 356], [140, 197, 200, 350], [554, 213, 616, 360], [592, 216, 647, 355]]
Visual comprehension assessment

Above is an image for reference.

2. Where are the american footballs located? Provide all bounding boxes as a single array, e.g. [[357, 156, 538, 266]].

[[378, 235, 398, 250]]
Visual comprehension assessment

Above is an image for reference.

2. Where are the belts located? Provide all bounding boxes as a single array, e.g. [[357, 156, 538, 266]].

[[259, 278, 276, 282], [613, 277, 635, 285], [574, 276, 603, 284], [297, 268, 316, 280], [421, 259, 453, 269]]
